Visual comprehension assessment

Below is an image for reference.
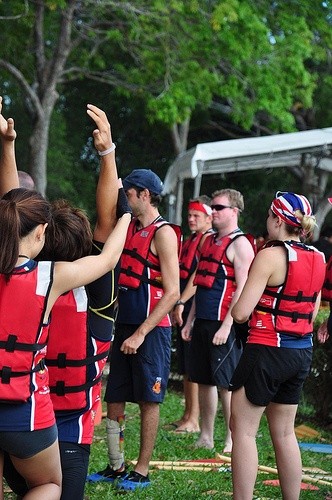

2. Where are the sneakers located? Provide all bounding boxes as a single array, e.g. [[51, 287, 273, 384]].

[[86, 464, 128, 482], [118, 470, 151, 489]]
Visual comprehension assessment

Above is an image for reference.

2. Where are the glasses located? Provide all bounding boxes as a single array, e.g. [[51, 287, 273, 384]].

[[276, 191, 288, 199], [210, 204, 243, 212]]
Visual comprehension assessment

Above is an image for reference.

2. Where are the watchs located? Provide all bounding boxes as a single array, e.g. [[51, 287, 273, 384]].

[[177, 300, 184, 305]]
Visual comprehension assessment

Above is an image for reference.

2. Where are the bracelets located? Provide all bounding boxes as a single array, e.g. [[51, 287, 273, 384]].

[[98, 143, 116, 156]]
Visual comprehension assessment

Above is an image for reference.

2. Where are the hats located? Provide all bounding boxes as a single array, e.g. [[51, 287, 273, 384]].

[[119, 168, 164, 195], [271, 195, 314, 227]]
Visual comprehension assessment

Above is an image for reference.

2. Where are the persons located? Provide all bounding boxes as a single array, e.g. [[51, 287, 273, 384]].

[[0, 96, 332, 500]]
